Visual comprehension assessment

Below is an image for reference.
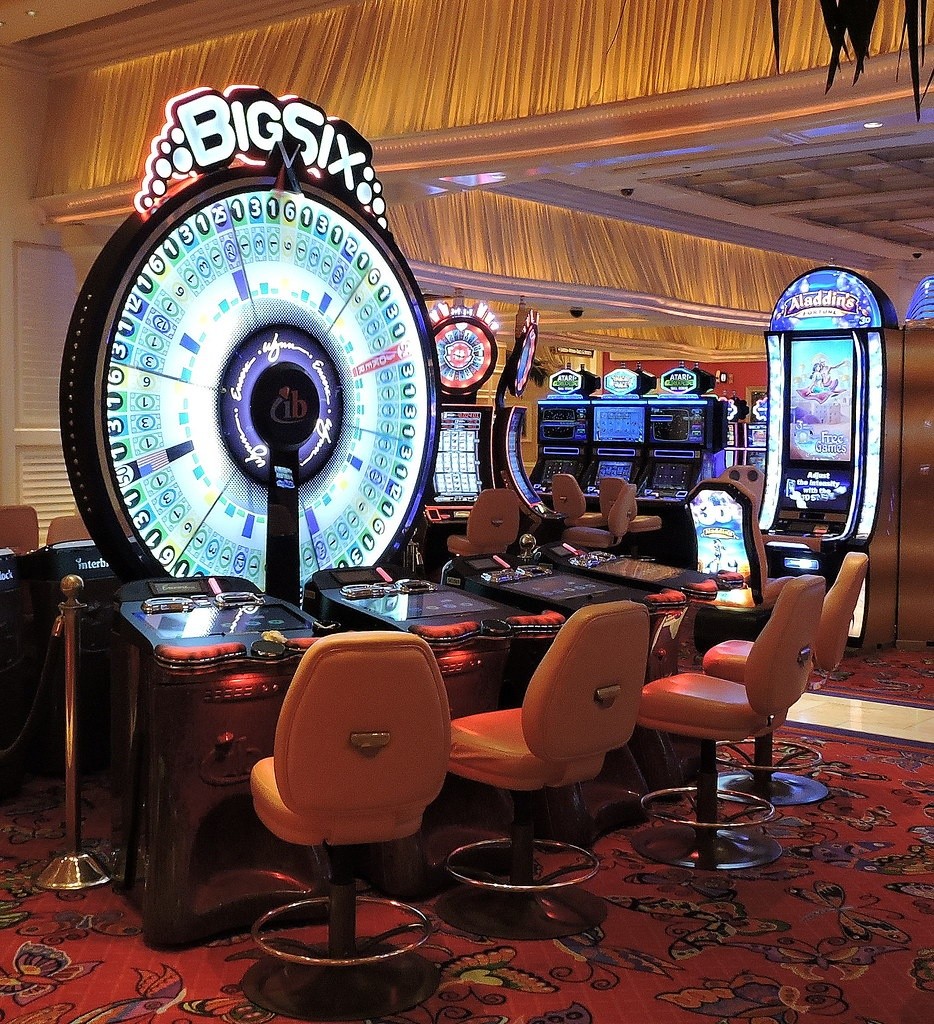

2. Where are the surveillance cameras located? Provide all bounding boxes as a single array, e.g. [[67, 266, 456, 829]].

[[913, 252, 922, 258], [569, 307, 584, 318], [621, 188, 633, 197]]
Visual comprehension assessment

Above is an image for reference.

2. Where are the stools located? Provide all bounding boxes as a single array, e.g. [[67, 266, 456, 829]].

[[639, 575, 827, 871], [244, 630, 454, 1024], [701, 552, 870, 807], [46, 514, 91, 545], [553, 473, 661, 552], [454, 602, 650, 941], [441, 489, 521, 582], [0, 504, 39, 555]]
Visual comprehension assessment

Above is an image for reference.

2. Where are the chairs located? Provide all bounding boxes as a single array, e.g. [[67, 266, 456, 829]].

[[719, 464, 765, 516], [685, 481, 795, 665]]
